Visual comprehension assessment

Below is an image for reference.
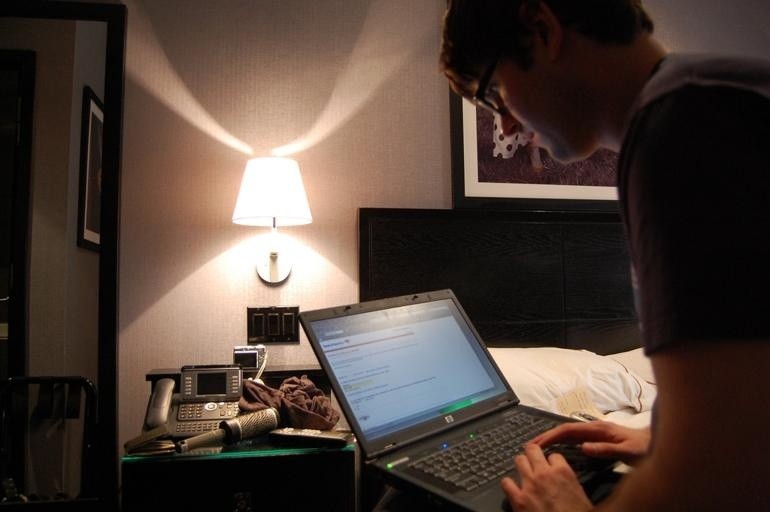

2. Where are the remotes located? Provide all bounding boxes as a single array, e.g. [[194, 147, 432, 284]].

[[268, 425, 350, 449]]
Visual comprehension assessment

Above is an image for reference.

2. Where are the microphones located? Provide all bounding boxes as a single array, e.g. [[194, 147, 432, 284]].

[[174, 407, 280, 452]]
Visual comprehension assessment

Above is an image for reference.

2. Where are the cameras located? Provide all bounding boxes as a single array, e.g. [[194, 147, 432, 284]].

[[233, 345, 266, 370]]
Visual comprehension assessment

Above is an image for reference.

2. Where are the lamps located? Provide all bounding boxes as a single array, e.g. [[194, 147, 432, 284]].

[[231, 157, 314, 285]]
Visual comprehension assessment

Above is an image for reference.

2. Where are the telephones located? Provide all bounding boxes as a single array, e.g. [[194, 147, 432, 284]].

[[146, 364, 243, 439]]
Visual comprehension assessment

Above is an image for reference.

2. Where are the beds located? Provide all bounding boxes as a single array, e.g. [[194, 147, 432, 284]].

[[355, 206, 657, 512]]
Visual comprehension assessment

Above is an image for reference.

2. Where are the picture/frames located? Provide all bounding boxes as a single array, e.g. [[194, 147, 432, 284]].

[[447, 81, 621, 210], [76, 84, 104, 255]]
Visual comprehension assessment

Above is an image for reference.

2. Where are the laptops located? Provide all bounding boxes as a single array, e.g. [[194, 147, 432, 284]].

[[299, 286, 622, 512]]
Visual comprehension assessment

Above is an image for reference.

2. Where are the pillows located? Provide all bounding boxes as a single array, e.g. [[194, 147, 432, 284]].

[[486, 346, 643, 418], [606, 346, 659, 413]]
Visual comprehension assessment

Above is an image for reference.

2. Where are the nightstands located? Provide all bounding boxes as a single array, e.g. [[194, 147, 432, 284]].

[[119, 438, 358, 512]]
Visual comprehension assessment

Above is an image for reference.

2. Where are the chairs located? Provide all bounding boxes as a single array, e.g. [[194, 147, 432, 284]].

[[0, 376, 108, 511]]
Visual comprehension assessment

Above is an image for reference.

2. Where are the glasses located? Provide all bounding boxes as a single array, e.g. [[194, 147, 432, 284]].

[[474, 56, 503, 120]]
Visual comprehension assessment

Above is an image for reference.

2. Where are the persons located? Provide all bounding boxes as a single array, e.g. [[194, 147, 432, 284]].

[[437, 1, 770, 512]]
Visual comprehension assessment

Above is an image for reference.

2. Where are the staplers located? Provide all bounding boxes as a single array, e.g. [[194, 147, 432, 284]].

[[123, 423, 176, 457]]
